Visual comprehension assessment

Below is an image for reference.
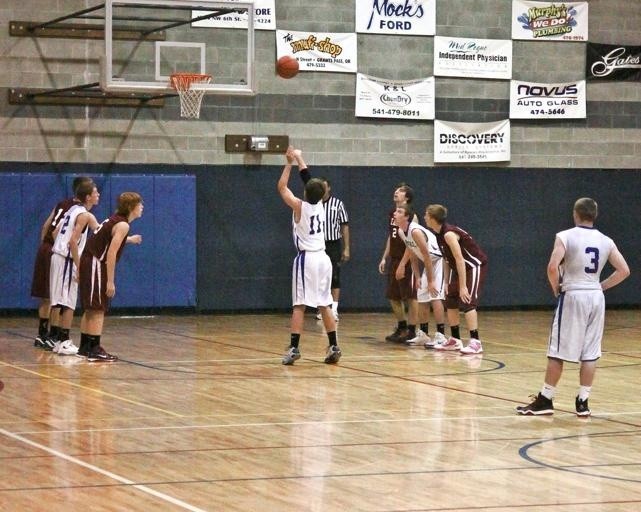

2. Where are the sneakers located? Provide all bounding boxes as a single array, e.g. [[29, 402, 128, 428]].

[[34, 335, 118, 362], [576, 395, 591, 416], [316, 310, 322, 320], [332, 312, 339, 321], [325, 345, 341, 363], [516, 392, 554, 415], [386, 326, 482, 354], [282, 348, 300, 365]]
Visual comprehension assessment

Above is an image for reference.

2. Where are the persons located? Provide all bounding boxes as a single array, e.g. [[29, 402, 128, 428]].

[[379, 183, 419, 345], [394, 205, 447, 349], [514, 198, 630, 416], [315, 179, 351, 322], [424, 206, 488, 356], [31, 175, 89, 349], [43, 182, 104, 357], [278, 146, 341, 365], [77, 191, 145, 362]]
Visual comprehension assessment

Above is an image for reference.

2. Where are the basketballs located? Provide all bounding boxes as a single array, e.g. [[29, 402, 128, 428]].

[[276, 55, 299, 79]]
